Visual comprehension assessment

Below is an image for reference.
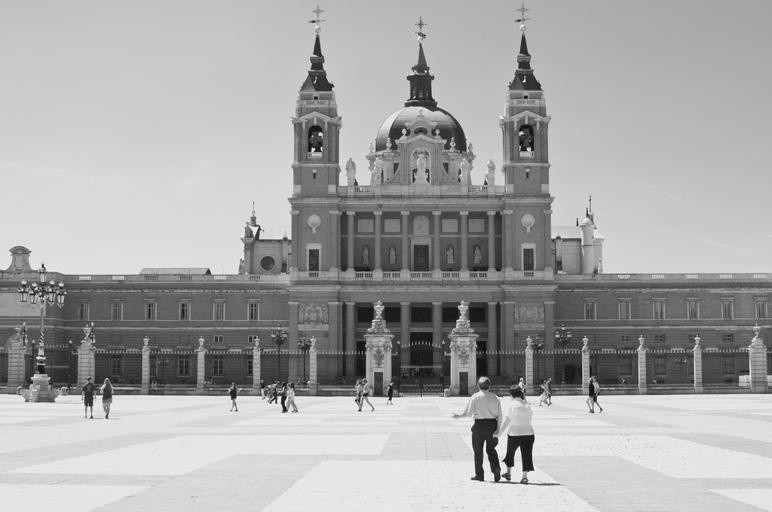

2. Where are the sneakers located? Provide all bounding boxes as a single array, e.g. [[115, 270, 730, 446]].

[[471, 477, 484, 481], [520, 478, 528, 484], [502, 473, 511, 480], [495, 473, 500, 482]]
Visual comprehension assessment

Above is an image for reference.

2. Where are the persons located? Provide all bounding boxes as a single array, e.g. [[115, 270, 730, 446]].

[[229, 382, 238, 411], [493, 384, 535, 483], [260, 378, 298, 413], [386, 381, 394, 405], [518, 376, 603, 413], [354, 378, 374, 412], [452, 377, 503, 482], [81, 377, 97, 419], [100, 378, 113, 419]]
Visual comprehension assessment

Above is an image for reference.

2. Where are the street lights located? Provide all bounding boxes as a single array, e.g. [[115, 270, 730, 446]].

[[15, 262, 68, 404], [269, 321, 288, 396], [674, 353, 688, 381], [297, 332, 311, 385], [530, 333, 544, 386], [554, 320, 574, 383]]
